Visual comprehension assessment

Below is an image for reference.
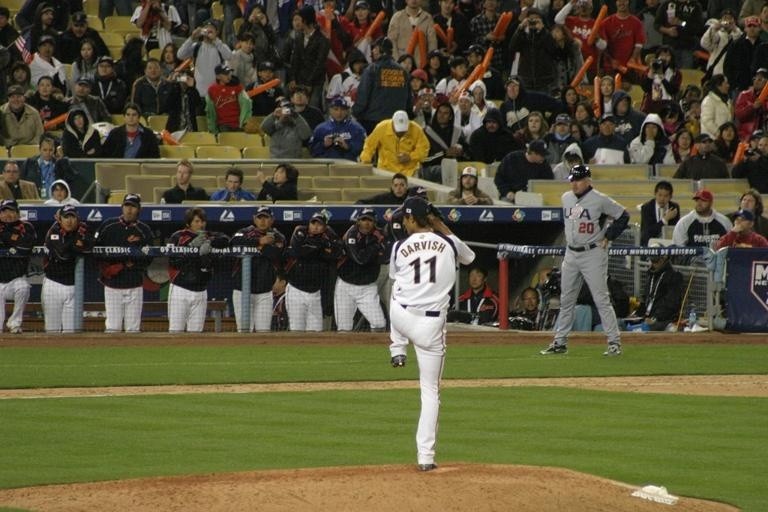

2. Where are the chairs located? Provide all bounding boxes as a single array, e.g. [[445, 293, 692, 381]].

[[0, 1, 767, 209]]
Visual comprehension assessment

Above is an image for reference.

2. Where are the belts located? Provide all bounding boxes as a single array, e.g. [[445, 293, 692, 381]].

[[401, 304, 439, 317], [568, 243, 596, 251]]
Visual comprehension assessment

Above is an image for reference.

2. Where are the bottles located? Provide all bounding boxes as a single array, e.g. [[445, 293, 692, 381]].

[[687, 305, 696, 325]]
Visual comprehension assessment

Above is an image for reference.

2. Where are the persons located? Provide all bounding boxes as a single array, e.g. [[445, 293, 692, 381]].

[[451, 267, 499, 324], [163, 159, 210, 204], [446, 165, 494, 204], [1, 194, 390, 332], [355, 174, 429, 205], [640, 181, 768, 333], [0, 137, 80, 207], [1, 0, 768, 178], [541, 164, 630, 354], [257, 162, 299, 200], [494, 140, 555, 204], [210, 164, 254, 200], [508, 287, 543, 330], [387, 196, 478, 473]]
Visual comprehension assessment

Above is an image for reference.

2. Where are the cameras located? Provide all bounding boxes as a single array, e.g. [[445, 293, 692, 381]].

[[652, 59, 666, 69], [743, 149, 754, 156], [721, 20, 729, 26], [282, 108, 291, 114], [333, 133, 340, 146], [256, 13, 262, 19], [201, 29, 208, 35], [528, 18, 536, 25]]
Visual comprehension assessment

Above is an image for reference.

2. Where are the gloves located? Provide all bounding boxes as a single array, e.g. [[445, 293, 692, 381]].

[[190, 234, 207, 247], [200, 239, 210, 256]]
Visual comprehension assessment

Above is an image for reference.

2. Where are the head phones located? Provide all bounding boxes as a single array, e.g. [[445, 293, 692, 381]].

[[379, 37, 388, 53]]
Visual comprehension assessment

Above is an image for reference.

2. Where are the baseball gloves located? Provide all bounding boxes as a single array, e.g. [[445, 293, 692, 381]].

[[425, 203, 445, 224]]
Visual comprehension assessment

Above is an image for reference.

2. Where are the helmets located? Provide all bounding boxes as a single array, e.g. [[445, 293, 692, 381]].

[[568, 165, 592, 182]]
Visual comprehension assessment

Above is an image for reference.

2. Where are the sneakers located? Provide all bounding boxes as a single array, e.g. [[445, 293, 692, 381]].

[[418, 464, 437, 470], [391, 355, 406, 367], [540, 341, 567, 355], [604, 342, 621, 355]]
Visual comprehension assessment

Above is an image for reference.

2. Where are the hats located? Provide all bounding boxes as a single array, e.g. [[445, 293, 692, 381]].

[[693, 190, 713, 202], [408, 185, 430, 202], [310, 212, 327, 225], [733, 209, 754, 221], [393, 198, 433, 215], [3, 0, 767, 160], [461, 166, 478, 179], [358, 208, 378, 222], [59, 204, 80, 217], [255, 205, 273, 219], [0, 199, 17, 212], [123, 193, 141, 209]]
